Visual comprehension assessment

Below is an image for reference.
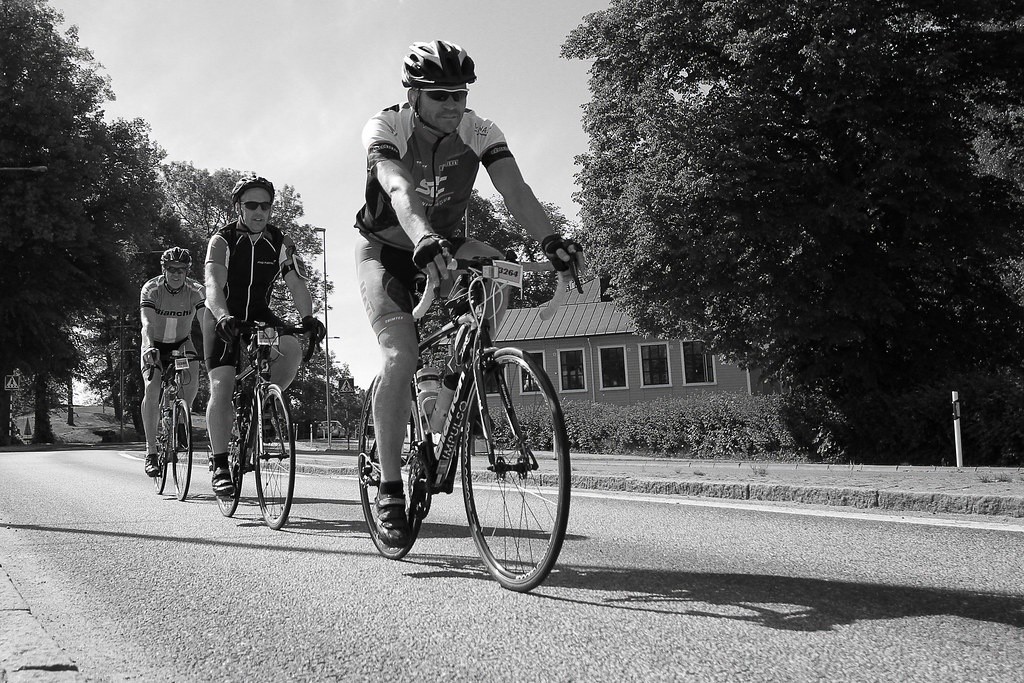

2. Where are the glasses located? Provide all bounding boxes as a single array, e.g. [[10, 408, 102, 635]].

[[413, 88, 470, 102], [164, 265, 187, 274], [240, 201, 272, 211]]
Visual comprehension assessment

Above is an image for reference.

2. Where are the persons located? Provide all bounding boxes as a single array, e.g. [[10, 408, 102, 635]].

[[203, 175, 326, 495], [139, 247, 206, 477], [352, 40, 588, 546]]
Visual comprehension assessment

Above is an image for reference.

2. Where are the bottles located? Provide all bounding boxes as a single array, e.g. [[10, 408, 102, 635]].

[[429, 371, 460, 432], [235, 389, 247, 423], [163, 407, 173, 451], [416, 361, 439, 430]]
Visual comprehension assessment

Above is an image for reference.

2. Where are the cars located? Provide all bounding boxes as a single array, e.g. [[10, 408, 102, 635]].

[[319, 421, 346, 438]]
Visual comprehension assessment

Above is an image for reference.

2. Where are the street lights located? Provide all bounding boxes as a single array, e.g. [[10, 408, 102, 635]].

[[313, 227, 331, 448]]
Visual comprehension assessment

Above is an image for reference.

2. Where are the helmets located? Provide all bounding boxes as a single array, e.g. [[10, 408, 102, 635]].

[[160, 247, 192, 268], [402, 39, 477, 88], [231, 175, 275, 203]]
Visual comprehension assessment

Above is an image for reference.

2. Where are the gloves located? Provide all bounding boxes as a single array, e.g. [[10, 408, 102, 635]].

[[214, 315, 252, 345], [302, 316, 327, 344]]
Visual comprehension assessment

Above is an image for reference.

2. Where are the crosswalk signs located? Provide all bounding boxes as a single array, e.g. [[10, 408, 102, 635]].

[[4, 375, 20, 391], [338, 377, 354, 394]]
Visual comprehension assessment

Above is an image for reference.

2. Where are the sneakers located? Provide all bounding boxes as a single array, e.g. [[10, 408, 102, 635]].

[[473, 415, 495, 435], [370, 494, 411, 549], [261, 403, 276, 444], [211, 466, 235, 496], [174, 423, 188, 448], [145, 454, 160, 477]]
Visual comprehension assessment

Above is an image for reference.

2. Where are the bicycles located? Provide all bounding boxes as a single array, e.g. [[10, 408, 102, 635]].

[[149, 350, 203, 501], [357, 248, 585, 592], [207, 317, 323, 530]]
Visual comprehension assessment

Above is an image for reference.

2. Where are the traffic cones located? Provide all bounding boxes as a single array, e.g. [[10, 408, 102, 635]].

[[22, 418, 33, 440]]
